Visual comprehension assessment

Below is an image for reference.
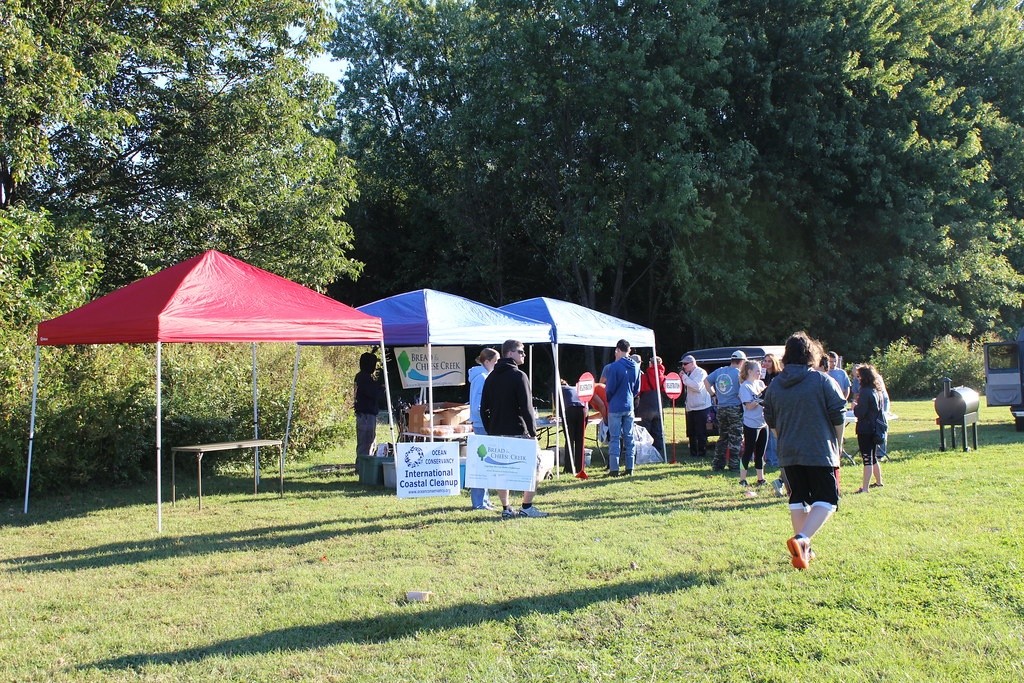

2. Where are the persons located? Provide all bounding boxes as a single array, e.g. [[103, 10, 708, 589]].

[[764, 331, 846, 570], [583, 340, 888, 496], [354, 352, 386, 474], [467, 348, 500, 513], [481, 339, 548, 519], [555, 378, 585, 474]]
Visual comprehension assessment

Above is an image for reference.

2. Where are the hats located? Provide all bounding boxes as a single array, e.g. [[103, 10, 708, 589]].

[[731, 350, 746, 359], [650, 356, 662, 364], [679, 355, 695, 364], [630, 354, 644, 363]]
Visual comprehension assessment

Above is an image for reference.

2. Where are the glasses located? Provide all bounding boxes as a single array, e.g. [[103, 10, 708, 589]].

[[507, 350, 526, 354]]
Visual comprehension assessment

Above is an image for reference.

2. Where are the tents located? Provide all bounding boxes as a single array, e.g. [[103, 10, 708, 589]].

[[492, 296, 668, 465], [25, 249, 395, 532], [280, 287, 575, 488]]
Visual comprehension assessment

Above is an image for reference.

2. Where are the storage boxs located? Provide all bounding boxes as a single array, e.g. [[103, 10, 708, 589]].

[[359, 455, 467, 491], [409, 399, 471, 434], [547, 447, 593, 467]]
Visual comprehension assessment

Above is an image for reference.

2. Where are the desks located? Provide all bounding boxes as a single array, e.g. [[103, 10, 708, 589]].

[[173, 439, 282, 512], [537, 417, 642, 466], [844, 414, 898, 467], [406, 435, 467, 443]]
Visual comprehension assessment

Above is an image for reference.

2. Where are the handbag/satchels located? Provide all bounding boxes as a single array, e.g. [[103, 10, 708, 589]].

[[873, 408, 888, 445]]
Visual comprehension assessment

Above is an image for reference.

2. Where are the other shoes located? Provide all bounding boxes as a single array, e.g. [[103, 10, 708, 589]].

[[483, 506, 499, 514], [757, 479, 770, 486], [622, 470, 633, 476], [869, 483, 884, 487], [740, 480, 750, 486], [604, 471, 617, 477], [854, 488, 863, 493]]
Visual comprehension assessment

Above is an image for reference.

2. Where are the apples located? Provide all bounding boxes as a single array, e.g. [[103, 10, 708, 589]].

[[420, 427, 443, 436]]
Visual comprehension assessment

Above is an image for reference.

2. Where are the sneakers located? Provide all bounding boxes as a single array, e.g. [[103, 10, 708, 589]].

[[787, 535, 813, 569], [771, 479, 783, 498], [519, 505, 549, 518], [502, 509, 519, 518]]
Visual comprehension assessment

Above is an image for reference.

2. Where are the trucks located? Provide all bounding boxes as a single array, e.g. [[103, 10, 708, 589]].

[[680, 345, 843, 437], [984, 326, 1024, 432]]
